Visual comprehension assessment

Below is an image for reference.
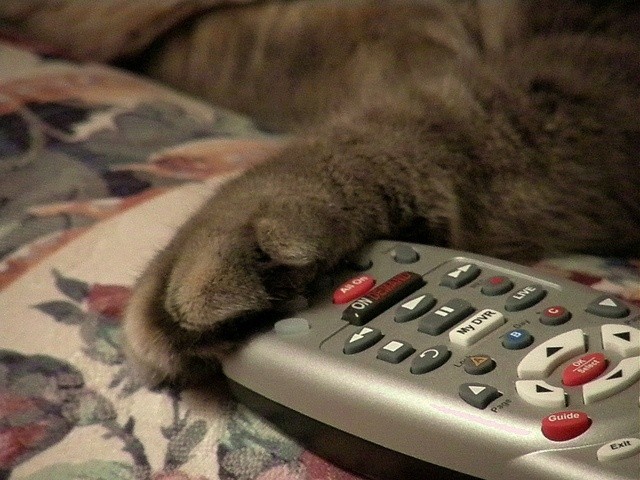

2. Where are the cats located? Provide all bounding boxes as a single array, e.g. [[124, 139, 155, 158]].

[[0, 1, 639, 395]]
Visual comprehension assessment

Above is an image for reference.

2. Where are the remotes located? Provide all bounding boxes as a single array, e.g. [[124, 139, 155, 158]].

[[221, 238, 639, 479]]
